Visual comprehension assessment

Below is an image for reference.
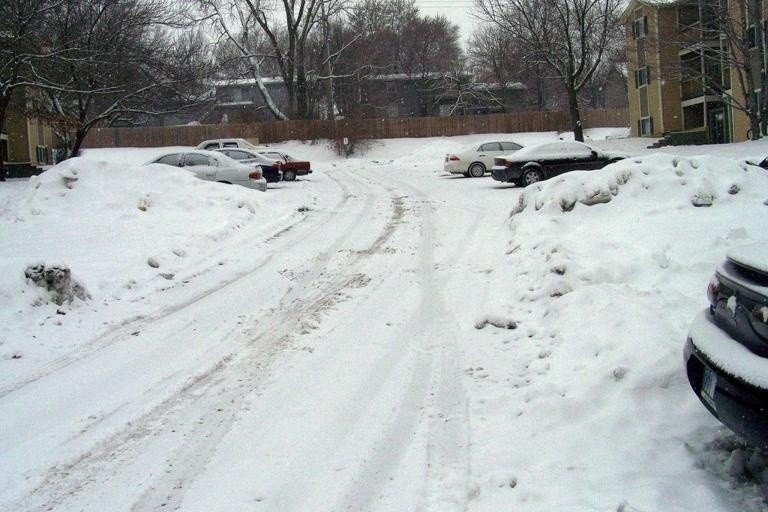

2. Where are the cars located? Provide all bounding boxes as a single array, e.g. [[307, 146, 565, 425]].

[[443, 140, 628, 187], [140, 138, 313, 192], [682, 243, 768, 455]]
